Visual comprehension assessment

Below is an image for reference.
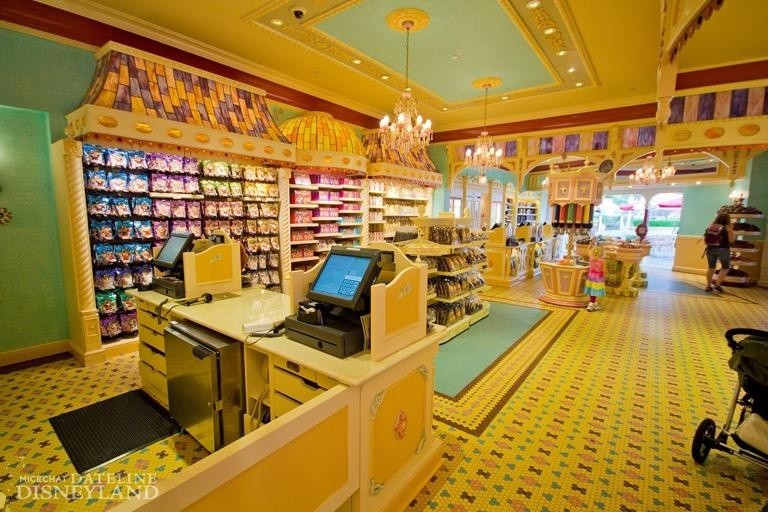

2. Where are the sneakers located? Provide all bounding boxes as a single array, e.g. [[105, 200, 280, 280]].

[[587, 303, 598, 311], [594, 302, 603, 310]]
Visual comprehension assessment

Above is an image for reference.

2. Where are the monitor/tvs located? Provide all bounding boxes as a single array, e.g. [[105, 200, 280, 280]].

[[150, 231, 195, 270], [307, 248, 381, 310]]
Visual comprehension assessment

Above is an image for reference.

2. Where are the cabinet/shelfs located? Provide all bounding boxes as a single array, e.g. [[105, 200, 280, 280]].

[[290, 166, 369, 314], [131, 298, 171, 413], [369, 177, 433, 244], [711, 211, 764, 287], [243, 345, 342, 435], [485, 227, 570, 288], [506, 197, 539, 237], [539, 254, 591, 308], [575, 238, 651, 298], [398, 217, 490, 346]]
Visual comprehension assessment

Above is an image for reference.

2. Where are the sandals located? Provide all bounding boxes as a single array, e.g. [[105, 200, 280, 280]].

[[705, 286, 713, 292], [714, 285, 723, 292]]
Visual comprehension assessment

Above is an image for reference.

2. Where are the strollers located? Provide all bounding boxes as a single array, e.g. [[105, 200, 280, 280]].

[[689, 327, 767, 473]]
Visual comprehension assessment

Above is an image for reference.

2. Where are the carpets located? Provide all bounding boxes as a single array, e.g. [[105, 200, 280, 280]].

[[48, 388, 181, 474]]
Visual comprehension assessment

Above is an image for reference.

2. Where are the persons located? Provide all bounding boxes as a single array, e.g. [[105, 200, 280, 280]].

[[584, 246, 606, 311], [704, 206, 736, 293]]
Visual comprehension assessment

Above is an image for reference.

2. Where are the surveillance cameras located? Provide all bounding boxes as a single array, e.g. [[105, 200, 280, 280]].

[[289, 6, 307, 19]]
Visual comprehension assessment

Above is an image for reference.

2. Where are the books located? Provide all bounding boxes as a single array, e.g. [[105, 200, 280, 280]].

[[361, 313, 370, 350]]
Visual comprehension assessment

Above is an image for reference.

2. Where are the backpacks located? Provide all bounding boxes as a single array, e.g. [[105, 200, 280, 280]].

[[703, 223, 726, 246]]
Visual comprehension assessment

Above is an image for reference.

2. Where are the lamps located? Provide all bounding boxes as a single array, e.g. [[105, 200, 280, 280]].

[[378, 22, 434, 153], [461, 82, 506, 186], [661, 154, 675, 180], [628, 155, 661, 186]]
